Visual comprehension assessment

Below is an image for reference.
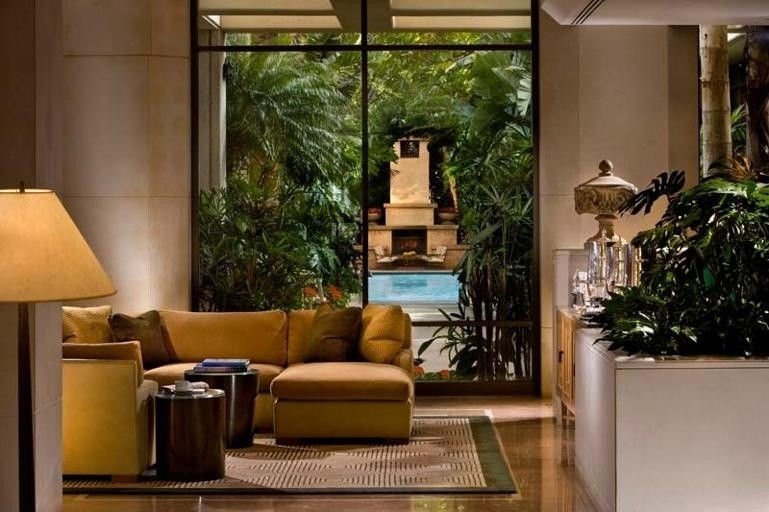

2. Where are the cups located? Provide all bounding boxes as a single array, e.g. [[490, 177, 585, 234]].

[[175, 380, 189, 391]]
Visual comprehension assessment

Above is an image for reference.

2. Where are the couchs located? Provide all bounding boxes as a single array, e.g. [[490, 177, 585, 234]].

[[60, 314, 416, 482]]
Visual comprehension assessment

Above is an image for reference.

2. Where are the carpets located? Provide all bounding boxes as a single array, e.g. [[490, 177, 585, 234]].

[[61, 415, 521, 495]]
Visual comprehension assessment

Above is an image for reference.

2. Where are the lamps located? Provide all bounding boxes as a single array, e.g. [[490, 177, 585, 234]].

[[0, 179, 118, 512]]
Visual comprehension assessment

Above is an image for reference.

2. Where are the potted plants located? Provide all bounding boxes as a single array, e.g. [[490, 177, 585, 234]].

[[368, 208, 382, 226], [437, 207, 457, 225]]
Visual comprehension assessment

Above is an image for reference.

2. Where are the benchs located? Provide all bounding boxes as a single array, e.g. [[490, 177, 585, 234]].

[[373, 245, 399, 269], [421, 245, 448, 270]]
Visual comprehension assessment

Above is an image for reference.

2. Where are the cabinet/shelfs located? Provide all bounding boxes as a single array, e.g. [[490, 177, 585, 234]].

[[554, 306, 603, 428]]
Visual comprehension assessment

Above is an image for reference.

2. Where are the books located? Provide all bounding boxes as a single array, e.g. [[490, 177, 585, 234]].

[[160, 381, 209, 394], [194, 358, 251, 373]]
